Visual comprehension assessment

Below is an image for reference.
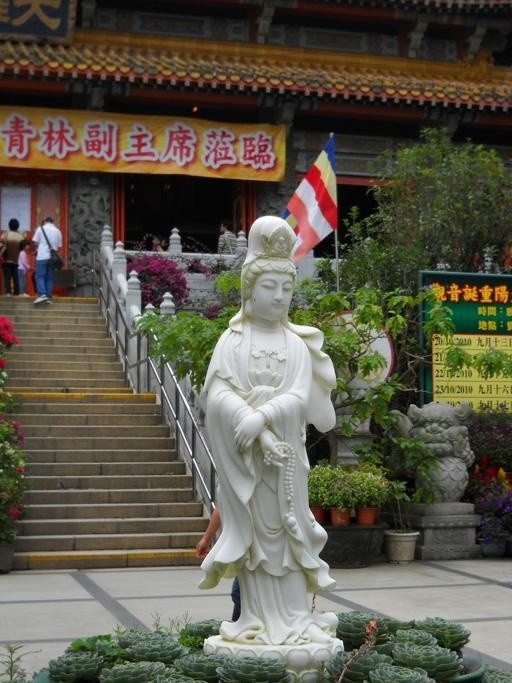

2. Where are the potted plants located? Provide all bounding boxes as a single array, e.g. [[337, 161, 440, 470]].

[[305, 431, 447, 566]]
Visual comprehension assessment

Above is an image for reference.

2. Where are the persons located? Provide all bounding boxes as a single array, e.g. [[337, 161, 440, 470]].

[[217, 223, 237, 255], [0, 217, 63, 305], [197, 214, 338, 645], [198, 504, 240, 621]]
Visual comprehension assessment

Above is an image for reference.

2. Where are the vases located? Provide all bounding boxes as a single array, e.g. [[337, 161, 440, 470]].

[[0, 541, 15, 572], [479, 537, 508, 558]]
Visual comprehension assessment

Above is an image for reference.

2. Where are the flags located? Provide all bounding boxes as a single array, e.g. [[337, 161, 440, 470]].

[[283, 135, 338, 259]]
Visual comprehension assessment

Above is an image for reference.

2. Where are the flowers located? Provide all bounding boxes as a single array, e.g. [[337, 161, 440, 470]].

[[125, 251, 193, 310], [467, 449, 512, 557], [0, 310, 29, 540]]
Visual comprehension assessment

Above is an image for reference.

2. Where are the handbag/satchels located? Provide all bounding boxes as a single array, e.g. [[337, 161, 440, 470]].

[[50, 250, 64, 268], [0, 243, 9, 258]]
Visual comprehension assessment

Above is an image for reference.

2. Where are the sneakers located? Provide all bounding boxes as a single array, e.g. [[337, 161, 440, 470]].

[[34, 294, 52, 306], [3, 292, 33, 297]]
